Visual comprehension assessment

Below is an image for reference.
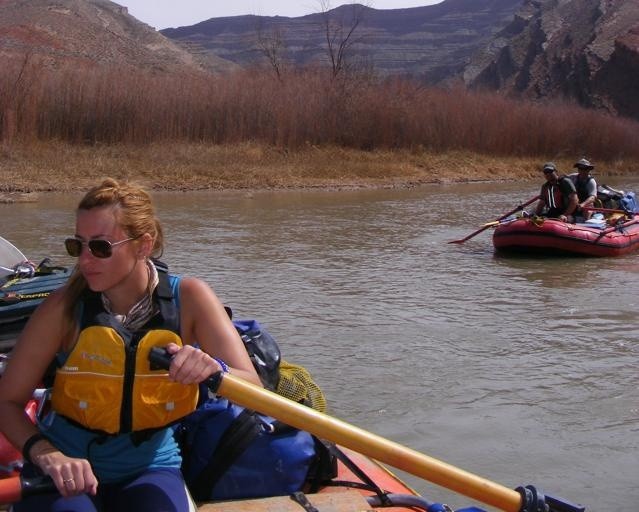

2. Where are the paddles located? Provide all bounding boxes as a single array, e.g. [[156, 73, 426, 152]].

[[447, 195, 540, 245]]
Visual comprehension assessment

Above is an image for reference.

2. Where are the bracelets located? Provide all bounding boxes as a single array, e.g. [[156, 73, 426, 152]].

[[213, 356, 234, 375], [21, 433, 60, 466]]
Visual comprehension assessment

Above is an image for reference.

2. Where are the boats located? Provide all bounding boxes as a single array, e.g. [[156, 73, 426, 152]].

[[492, 209, 639, 255], [0, 238, 480, 511]]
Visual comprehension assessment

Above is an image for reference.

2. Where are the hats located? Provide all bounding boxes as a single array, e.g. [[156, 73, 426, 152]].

[[573, 159, 596, 170], [543, 163, 557, 172]]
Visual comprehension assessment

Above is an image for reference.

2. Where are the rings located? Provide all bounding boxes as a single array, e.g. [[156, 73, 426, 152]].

[[62, 477, 75, 483]]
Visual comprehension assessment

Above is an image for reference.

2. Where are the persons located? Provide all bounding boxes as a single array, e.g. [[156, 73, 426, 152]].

[[1, 176, 265, 512], [535, 158, 598, 223]]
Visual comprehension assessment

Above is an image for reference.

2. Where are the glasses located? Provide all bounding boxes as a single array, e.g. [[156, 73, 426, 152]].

[[64, 237, 138, 259]]
